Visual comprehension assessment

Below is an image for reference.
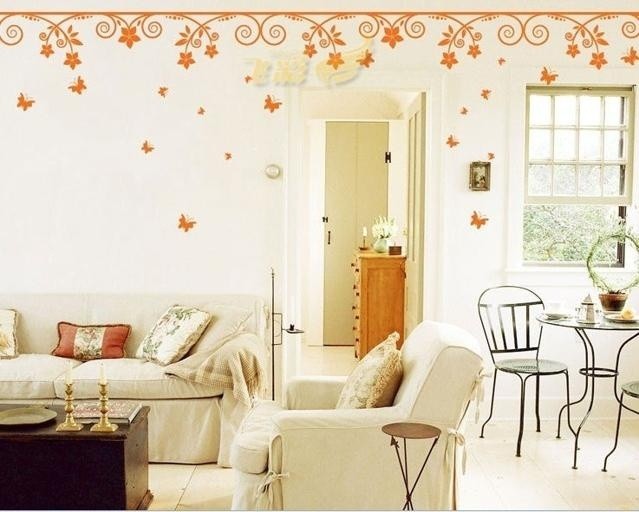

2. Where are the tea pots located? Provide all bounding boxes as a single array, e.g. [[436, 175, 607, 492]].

[[575, 302, 598, 323]]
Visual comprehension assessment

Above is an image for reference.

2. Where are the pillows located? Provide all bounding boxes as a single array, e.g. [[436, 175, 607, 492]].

[[330, 330, 410, 410], [1, 308, 20, 358], [54, 321, 132, 360], [132, 301, 212, 367]]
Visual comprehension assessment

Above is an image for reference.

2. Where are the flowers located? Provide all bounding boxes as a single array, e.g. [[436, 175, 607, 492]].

[[372, 217, 393, 238]]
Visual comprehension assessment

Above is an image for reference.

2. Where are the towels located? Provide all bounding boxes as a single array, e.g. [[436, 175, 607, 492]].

[[578, 293, 595, 322]]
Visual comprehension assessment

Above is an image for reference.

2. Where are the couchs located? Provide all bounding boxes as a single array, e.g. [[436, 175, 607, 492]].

[[1, 289, 273, 467]]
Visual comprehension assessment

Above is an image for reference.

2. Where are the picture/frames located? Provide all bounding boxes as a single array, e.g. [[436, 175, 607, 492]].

[[470, 162, 491, 191]]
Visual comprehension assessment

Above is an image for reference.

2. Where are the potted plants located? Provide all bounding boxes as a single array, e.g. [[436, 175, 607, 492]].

[[585, 214, 639, 313]]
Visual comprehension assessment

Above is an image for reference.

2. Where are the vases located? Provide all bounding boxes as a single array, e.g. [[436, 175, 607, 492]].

[[374, 236, 387, 251]]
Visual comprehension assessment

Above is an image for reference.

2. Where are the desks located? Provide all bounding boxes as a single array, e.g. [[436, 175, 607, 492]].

[[534, 304, 639, 472], [380, 421, 440, 509]]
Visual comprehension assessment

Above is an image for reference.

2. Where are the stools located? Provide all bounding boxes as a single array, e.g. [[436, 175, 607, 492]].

[[602, 382, 639, 473]]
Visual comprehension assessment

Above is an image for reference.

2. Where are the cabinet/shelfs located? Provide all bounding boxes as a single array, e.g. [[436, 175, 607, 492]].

[[352, 251, 407, 362]]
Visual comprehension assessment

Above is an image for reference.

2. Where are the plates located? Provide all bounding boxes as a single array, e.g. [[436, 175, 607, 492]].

[[604, 313, 639, 323], [0, 407, 58, 426]]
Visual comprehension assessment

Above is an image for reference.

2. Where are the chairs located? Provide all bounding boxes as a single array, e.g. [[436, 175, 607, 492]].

[[226, 318, 488, 510], [477, 283, 577, 457]]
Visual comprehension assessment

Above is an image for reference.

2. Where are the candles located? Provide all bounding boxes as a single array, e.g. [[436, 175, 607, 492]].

[[63, 358, 73, 382], [361, 224, 367, 246], [97, 361, 107, 382]]
